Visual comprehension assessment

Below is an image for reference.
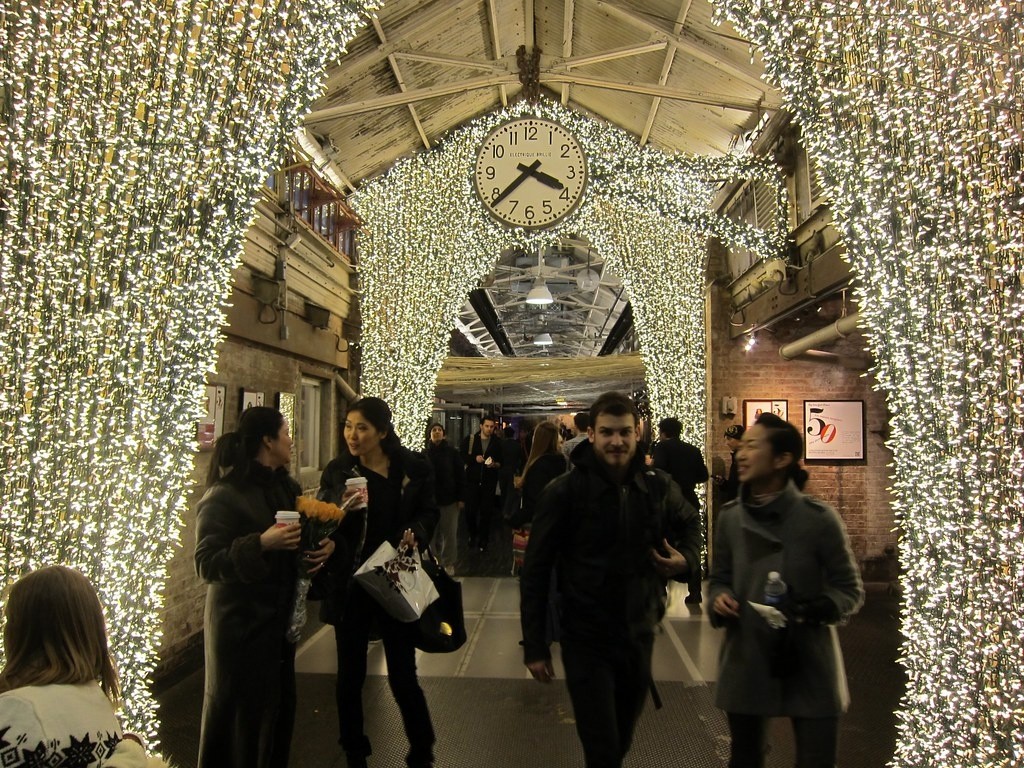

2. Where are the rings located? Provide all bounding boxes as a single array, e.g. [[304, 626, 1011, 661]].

[[320, 562, 324, 566]]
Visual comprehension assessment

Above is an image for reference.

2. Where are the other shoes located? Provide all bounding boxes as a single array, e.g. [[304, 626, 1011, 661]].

[[478, 537, 486, 553], [467, 535, 477, 545], [685, 593, 702, 603]]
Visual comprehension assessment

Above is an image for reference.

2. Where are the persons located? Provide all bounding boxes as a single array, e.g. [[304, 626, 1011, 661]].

[[315, 397, 441, 768], [0, 566, 124, 768], [518, 392, 701, 768], [706, 413, 865, 768], [421, 412, 709, 605], [193, 407, 335, 768]]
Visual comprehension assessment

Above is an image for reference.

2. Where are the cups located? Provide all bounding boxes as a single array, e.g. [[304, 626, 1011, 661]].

[[345, 477, 368, 510], [484, 456, 495, 467], [274, 511, 301, 531]]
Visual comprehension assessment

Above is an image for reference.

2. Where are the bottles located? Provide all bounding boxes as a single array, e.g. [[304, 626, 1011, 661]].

[[763, 572, 790, 616]]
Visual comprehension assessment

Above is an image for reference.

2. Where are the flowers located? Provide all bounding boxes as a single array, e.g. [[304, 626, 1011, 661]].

[[194, 382, 227, 452]]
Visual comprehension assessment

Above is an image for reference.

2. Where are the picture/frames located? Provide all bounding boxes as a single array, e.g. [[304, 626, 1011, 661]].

[[743, 399, 788, 431], [803, 400, 866, 461]]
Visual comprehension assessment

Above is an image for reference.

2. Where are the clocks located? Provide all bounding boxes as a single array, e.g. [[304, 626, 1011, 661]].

[[473, 118, 588, 231]]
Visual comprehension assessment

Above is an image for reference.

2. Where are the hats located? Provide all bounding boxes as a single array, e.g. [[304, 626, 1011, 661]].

[[723, 425, 745, 440]]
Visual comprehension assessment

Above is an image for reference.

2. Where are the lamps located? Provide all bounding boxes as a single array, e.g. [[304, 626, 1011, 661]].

[[525, 243, 553, 305], [576, 248, 600, 291], [534, 323, 553, 345]]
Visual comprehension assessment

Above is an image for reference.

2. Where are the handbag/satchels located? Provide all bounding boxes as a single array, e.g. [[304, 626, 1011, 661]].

[[353, 539, 440, 623], [441, 432, 477, 502], [512, 525, 531, 577], [416, 547, 467, 653]]
